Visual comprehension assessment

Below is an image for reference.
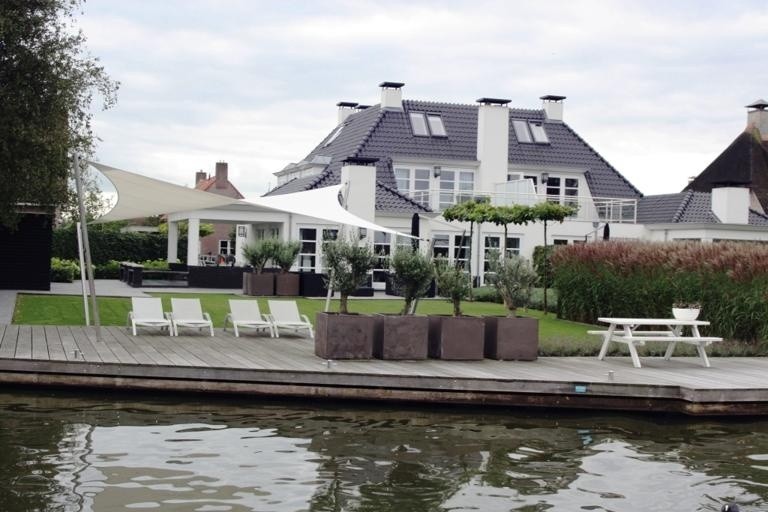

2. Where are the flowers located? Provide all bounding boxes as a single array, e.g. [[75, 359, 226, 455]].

[[672, 299, 703, 309]]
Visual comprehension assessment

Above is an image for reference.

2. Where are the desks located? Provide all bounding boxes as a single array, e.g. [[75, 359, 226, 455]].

[[597, 317, 713, 369]]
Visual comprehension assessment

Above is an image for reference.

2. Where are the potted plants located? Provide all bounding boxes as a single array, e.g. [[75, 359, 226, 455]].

[[482, 235, 546, 360], [429, 254, 488, 361], [313, 226, 386, 360], [241, 239, 303, 298], [373, 239, 437, 359]]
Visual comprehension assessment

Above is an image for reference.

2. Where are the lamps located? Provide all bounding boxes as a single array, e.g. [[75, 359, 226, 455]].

[[540, 172, 550, 184], [434, 165, 442, 178]]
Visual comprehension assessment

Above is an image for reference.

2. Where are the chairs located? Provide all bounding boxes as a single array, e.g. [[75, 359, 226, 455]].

[[125, 296, 174, 337], [223, 297, 275, 339], [262, 299, 317, 339], [166, 298, 215, 337]]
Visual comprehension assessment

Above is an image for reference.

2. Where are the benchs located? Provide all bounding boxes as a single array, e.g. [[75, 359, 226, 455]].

[[610, 335, 724, 346], [585, 330, 684, 336]]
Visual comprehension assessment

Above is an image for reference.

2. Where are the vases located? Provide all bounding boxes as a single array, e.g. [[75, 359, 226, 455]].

[[672, 307, 700, 320]]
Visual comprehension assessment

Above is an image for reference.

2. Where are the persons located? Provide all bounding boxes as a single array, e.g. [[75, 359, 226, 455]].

[[207, 251, 236, 267]]
